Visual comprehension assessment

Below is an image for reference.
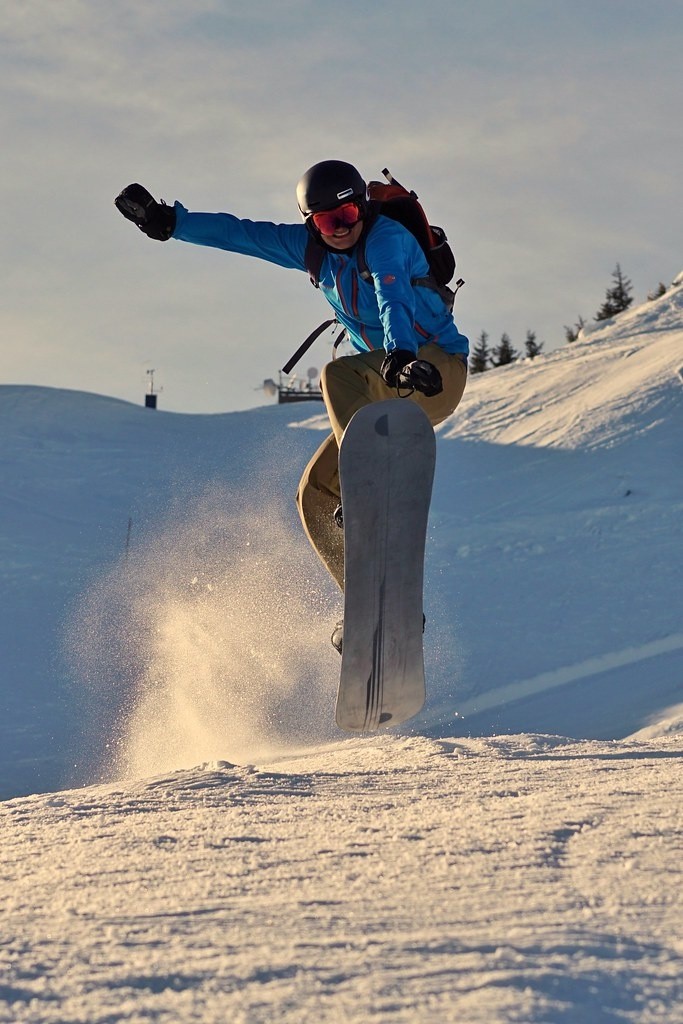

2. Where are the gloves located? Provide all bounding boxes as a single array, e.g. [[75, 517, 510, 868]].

[[379, 350, 443, 398], [114, 183, 177, 242]]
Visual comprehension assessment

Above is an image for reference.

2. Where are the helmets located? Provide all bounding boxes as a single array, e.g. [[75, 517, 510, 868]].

[[296, 160, 371, 241]]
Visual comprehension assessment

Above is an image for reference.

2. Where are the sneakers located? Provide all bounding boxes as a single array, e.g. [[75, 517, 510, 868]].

[[330, 612, 426, 655], [333, 502, 344, 529]]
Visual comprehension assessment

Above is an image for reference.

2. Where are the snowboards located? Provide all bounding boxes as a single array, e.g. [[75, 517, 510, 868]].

[[333, 395, 438, 734]]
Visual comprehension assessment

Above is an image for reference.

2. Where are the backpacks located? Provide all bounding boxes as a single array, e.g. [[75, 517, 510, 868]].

[[304, 168, 466, 313]]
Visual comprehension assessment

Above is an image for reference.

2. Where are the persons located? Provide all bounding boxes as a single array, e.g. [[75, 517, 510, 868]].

[[114, 159, 469, 652]]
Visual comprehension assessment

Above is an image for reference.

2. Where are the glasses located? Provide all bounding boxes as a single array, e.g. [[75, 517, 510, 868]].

[[297, 183, 370, 236]]
